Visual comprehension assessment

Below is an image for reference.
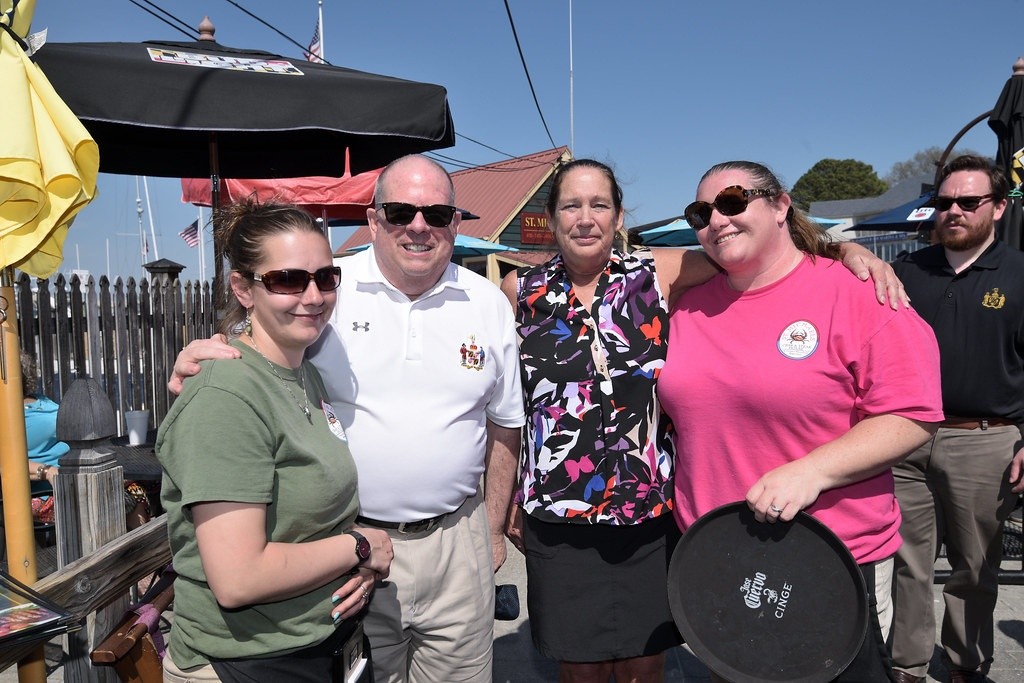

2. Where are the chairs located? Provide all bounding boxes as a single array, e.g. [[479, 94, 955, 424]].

[[0, 481, 55, 563]]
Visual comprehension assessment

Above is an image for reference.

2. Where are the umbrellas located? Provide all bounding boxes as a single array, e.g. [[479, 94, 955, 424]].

[[345, 234, 519, 256], [0, 0, 100, 683], [181, 148, 385, 232], [988, 58, 1024, 250], [28, 16, 456, 310], [638, 216, 847, 245], [842, 186, 936, 233]]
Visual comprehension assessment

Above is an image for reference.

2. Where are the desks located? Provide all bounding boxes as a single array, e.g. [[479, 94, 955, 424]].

[[109, 428, 173, 633]]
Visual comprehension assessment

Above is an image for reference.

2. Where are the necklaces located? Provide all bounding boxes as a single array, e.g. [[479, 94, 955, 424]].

[[244, 330, 311, 418]]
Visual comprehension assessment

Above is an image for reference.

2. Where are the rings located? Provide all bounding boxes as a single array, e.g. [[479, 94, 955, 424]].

[[360, 585, 369, 604], [771, 504, 784, 513]]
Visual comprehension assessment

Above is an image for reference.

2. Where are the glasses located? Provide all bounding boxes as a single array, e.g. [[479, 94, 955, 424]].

[[684, 185, 780, 231], [930, 194, 993, 212], [237, 266, 341, 294], [375, 202, 457, 228]]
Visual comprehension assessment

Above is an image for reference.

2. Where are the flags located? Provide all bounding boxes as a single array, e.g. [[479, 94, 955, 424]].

[[303, 17, 320, 63], [178, 219, 198, 248]]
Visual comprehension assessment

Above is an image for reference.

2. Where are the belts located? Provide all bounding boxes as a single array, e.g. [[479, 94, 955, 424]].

[[939, 416, 1018, 429], [355, 512, 445, 534]]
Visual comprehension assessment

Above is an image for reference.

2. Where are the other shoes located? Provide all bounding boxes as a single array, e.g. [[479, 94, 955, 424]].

[[949, 669, 995, 683], [891, 669, 926, 683]]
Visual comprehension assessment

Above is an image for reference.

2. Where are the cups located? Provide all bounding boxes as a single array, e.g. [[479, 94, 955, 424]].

[[124, 410, 151, 445]]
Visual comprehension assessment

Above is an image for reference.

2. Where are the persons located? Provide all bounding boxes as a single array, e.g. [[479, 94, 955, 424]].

[[657, 161, 945, 683], [151, 198, 395, 683], [168, 154, 526, 683], [500, 158, 912, 683], [885, 155, 1024, 683]]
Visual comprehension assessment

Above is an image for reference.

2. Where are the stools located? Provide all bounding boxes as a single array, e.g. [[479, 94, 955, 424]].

[[90, 563, 180, 683]]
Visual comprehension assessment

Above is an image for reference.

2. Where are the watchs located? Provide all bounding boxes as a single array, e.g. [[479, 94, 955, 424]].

[[348, 530, 371, 563]]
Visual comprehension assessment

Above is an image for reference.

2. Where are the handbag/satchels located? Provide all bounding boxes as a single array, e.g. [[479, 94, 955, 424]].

[[208, 614, 375, 683]]
